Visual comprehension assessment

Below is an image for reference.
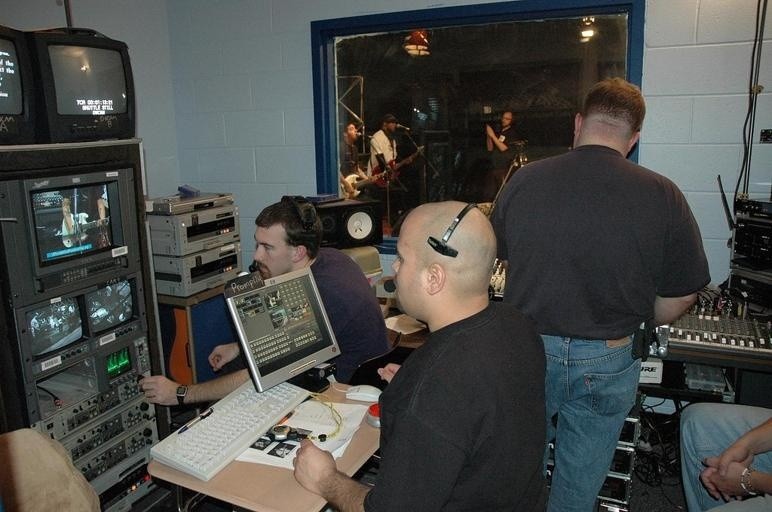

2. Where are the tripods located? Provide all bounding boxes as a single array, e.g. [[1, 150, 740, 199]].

[[487, 150, 529, 220]]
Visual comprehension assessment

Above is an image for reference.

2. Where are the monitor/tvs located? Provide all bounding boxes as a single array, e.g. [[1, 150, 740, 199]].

[[32, 31, 138, 144], [77, 279, 139, 337], [0, 23, 32, 145], [223, 268, 343, 393], [22, 170, 127, 278], [24, 296, 90, 361]]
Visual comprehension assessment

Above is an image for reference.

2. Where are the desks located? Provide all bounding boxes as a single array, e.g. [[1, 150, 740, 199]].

[[147, 382, 380, 512]]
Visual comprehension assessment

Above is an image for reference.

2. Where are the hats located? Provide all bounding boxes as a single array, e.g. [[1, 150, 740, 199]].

[[382, 115, 398, 122]]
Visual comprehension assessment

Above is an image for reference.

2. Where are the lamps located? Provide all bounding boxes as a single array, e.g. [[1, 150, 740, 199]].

[[403, 31, 430, 50], [406, 49, 431, 57]]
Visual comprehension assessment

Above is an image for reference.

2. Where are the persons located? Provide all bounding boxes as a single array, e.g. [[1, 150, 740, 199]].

[[139, 201, 388, 406], [62, 184, 106, 248], [339, 122, 369, 194], [367, 114, 398, 177], [680, 402, 772, 512], [490, 78, 711, 512], [292, 201, 547, 512], [485, 112, 518, 198]]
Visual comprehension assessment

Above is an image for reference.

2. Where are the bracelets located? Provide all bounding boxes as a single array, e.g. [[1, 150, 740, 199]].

[[740, 468, 761, 496]]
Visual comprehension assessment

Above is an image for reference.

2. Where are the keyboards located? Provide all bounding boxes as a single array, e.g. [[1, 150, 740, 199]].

[[150, 379, 308, 480]]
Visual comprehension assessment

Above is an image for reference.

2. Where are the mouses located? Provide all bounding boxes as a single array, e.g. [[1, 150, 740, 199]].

[[345, 384, 384, 402]]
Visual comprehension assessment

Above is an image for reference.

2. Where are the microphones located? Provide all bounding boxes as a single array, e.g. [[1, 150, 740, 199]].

[[357, 132, 374, 139], [395, 123, 410, 131], [249, 260, 257, 273], [384, 279, 397, 293]]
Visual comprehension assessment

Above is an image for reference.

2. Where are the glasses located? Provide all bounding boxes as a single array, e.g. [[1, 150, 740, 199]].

[[502, 117, 512, 121]]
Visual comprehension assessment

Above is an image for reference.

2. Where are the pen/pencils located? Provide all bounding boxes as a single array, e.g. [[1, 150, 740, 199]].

[[279, 410, 295, 425], [177, 407, 214, 434]]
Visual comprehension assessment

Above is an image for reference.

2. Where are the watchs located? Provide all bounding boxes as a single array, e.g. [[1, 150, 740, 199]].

[[176, 384, 188, 405]]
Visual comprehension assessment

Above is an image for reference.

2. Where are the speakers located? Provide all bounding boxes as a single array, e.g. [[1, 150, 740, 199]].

[[317, 198, 384, 250]]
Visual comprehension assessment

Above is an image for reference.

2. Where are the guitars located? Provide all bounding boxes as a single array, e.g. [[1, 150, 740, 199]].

[[61, 213, 111, 248], [372, 145, 425, 188], [341, 172, 388, 197]]
